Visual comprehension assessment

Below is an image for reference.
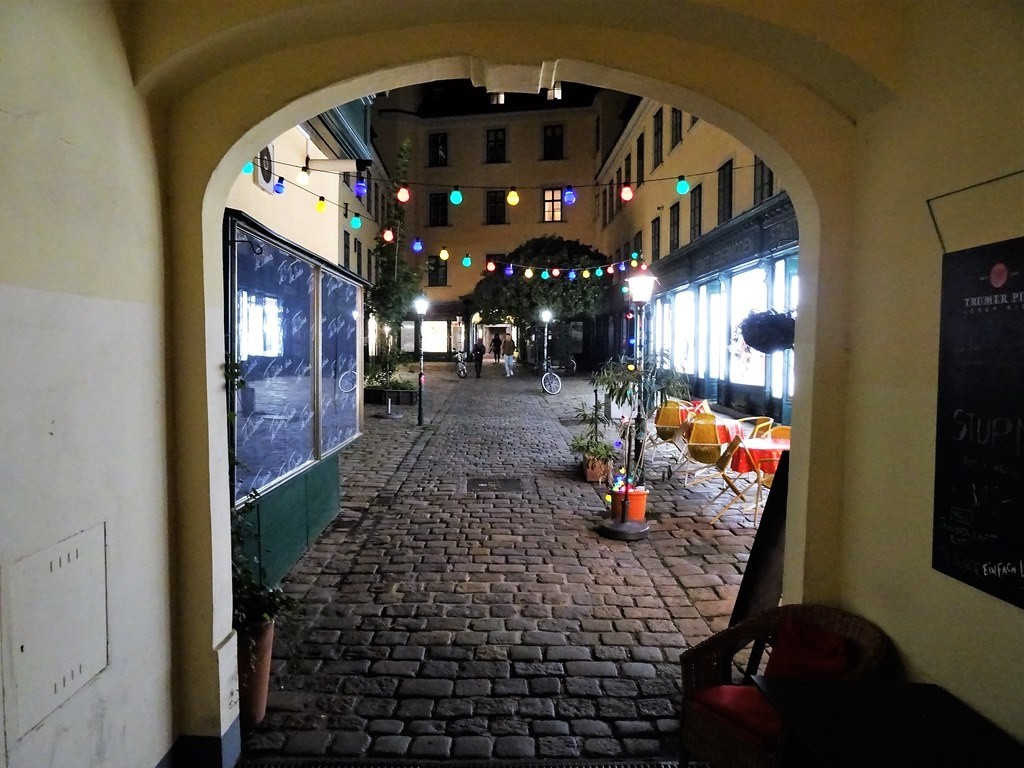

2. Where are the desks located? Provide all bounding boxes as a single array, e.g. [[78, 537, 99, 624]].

[[681, 407, 703, 422], [737, 436, 791, 528], [685, 419, 741, 476]]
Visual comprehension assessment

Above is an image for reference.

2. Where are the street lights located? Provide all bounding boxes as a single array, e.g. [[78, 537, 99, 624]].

[[542, 310, 552, 393], [412, 292, 429, 426], [625, 255, 656, 485], [456, 312, 463, 355]]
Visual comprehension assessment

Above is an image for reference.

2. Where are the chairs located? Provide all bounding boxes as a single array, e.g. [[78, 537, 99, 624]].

[[650, 398, 791, 526]]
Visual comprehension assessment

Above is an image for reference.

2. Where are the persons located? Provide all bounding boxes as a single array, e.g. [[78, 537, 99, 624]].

[[472, 338, 486, 379], [503, 333, 515, 377], [490, 333, 502, 363]]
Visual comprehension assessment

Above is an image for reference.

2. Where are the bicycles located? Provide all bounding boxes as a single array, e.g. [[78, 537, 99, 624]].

[[454, 350, 468, 379], [338, 365, 357, 393], [540, 357, 562, 395]]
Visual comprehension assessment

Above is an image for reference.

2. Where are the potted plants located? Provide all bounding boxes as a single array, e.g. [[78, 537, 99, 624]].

[[567, 369, 618, 484], [730, 304, 795, 365], [585, 348, 690, 523]]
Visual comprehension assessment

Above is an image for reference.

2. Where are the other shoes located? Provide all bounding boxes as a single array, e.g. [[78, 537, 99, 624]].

[[479, 375, 480, 378], [476, 376, 478, 379], [506, 374, 510, 377], [498, 361, 500, 363], [495, 362, 496, 363], [510, 370, 514, 375]]
